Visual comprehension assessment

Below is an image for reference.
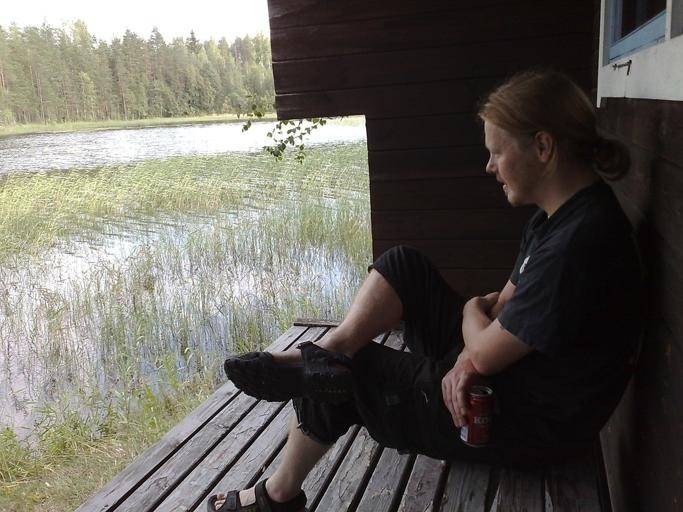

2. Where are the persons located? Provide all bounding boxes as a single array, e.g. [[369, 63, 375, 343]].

[[205, 70, 648, 512]]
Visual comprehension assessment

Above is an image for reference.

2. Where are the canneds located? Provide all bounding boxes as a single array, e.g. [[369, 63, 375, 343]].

[[459, 386, 495, 449]]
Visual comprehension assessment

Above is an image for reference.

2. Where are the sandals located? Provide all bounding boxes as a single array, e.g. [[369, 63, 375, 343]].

[[224, 342, 352, 403], [206, 478, 307, 510]]
